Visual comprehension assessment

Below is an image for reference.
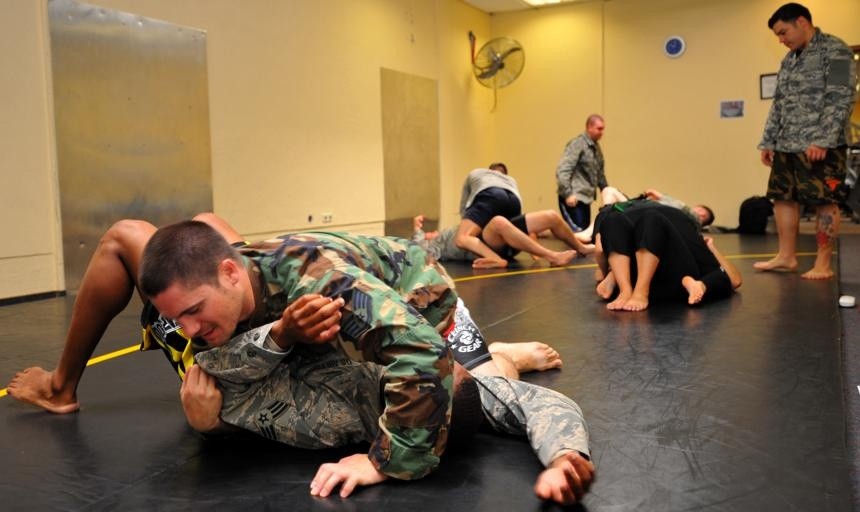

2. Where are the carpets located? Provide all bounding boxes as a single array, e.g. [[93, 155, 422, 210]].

[[0, 225, 855, 512]]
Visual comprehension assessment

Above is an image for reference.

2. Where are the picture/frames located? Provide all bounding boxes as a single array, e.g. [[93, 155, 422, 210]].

[[758, 72, 782, 100]]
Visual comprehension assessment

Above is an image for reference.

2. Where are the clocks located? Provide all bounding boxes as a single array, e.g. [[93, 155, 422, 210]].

[[662, 35, 686, 58]]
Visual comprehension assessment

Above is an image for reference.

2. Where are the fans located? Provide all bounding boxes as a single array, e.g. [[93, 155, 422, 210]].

[[469, 30, 526, 89]]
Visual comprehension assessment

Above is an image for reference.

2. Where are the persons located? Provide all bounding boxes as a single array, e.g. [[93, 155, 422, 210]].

[[556, 114, 608, 232], [411, 209, 595, 267], [8, 212, 597, 504], [140, 221, 563, 498], [575, 186, 743, 312], [454, 162, 539, 268], [753, 3, 857, 280]]
